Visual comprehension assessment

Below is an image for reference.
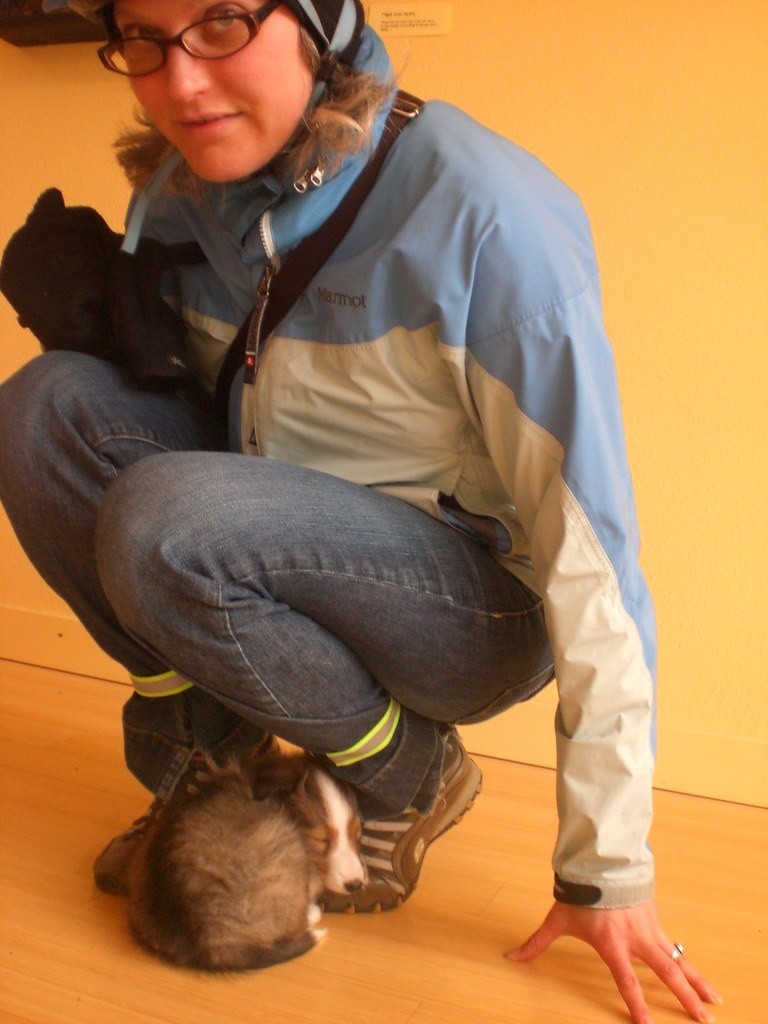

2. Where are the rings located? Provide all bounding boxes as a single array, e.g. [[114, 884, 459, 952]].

[[670, 943, 686, 963]]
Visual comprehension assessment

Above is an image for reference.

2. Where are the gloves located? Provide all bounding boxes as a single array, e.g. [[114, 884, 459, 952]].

[[0, 186, 205, 398]]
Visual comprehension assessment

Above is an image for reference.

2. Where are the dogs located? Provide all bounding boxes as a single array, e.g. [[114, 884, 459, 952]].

[[125, 746, 370, 974]]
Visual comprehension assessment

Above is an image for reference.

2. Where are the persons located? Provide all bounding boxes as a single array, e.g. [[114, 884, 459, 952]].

[[0, 0, 720, 1024]]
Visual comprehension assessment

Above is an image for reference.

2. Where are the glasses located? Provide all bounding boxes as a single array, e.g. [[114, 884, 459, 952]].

[[96, 0, 286, 76]]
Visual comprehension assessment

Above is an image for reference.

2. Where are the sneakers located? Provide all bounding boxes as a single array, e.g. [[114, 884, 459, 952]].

[[315, 720, 482, 913], [93, 750, 220, 896]]
